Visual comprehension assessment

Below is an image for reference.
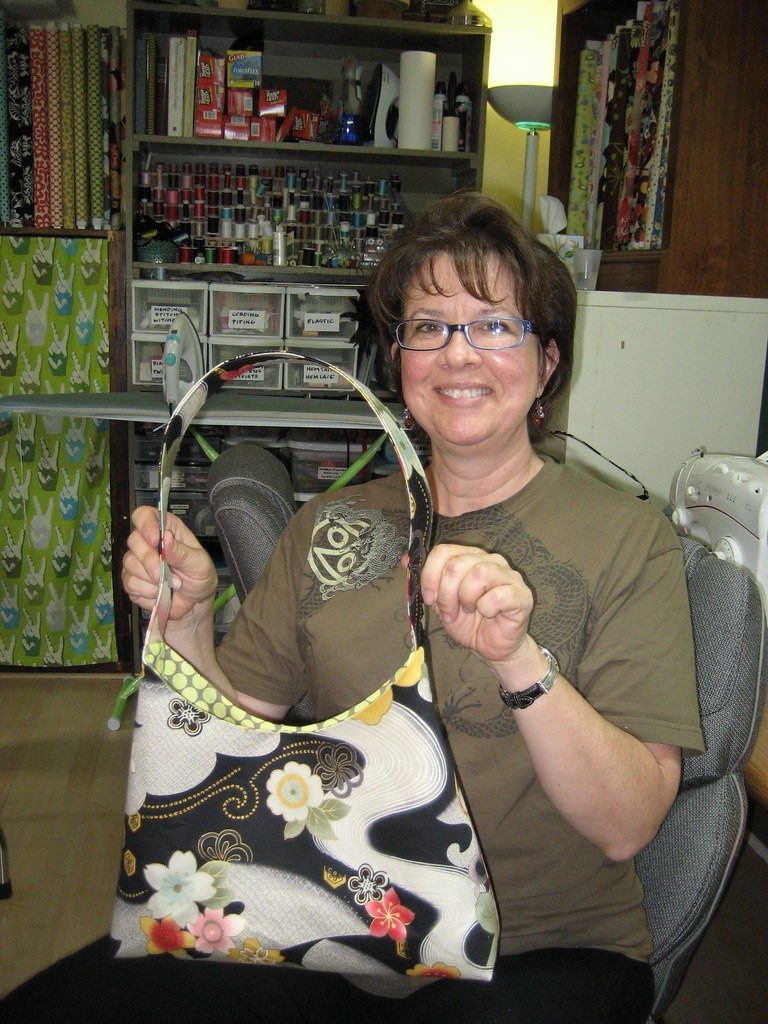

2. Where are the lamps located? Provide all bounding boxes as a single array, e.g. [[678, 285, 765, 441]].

[[484, 84, 556, 237]]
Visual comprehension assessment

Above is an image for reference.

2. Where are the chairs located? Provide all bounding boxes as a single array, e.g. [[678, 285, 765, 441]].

[[206, 443, 295, 600], [639, 536, 764, 1024]]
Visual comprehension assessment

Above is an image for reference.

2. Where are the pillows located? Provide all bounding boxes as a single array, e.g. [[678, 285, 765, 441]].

[[105, 677, 500, 983]]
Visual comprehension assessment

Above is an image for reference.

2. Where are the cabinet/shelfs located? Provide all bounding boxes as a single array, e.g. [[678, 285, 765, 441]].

[[128, 1, 491, 677], [554, 0, 767, 462]]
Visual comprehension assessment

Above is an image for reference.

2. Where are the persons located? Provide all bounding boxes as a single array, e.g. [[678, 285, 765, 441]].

[[122, 192, 706, 1024]]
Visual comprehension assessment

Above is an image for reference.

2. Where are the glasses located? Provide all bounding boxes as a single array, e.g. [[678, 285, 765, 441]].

[[391, 318, 535, 350]]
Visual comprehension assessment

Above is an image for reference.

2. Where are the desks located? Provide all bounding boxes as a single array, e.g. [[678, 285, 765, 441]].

[[0, 392, 420, 436]]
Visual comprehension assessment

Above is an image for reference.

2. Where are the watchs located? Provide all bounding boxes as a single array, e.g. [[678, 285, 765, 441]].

[[498, 645, 560, 710]]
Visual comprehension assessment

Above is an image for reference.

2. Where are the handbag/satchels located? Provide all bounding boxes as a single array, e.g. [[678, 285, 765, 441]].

[[109, 353, 502, 984]]
